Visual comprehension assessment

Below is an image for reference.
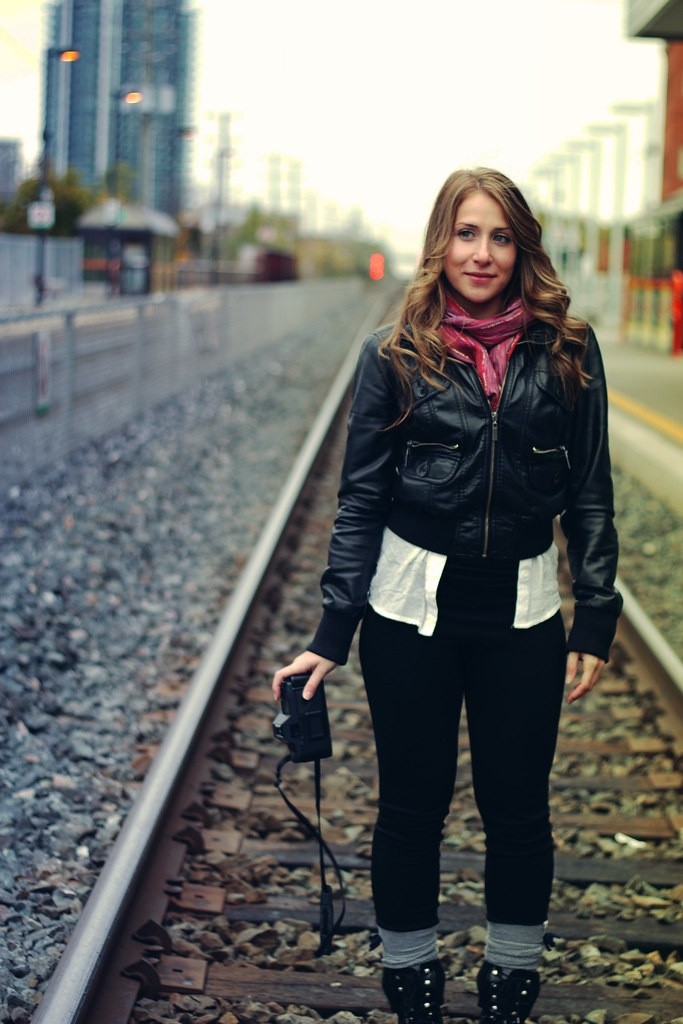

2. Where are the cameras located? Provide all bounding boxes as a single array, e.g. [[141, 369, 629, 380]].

[[270, 672, 333, 763]]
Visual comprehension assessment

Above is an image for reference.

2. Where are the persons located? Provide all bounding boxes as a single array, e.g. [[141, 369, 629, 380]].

[[271, 165, 623, 1024]]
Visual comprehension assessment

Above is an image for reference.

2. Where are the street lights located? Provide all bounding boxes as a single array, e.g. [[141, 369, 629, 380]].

[[32, 45, 81, 300], [110, 88, 142, 197]]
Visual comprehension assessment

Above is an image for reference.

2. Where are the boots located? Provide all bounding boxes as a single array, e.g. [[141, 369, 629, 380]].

[[475, 958, 540, 1024], [383, 955, 445, 1024]]
